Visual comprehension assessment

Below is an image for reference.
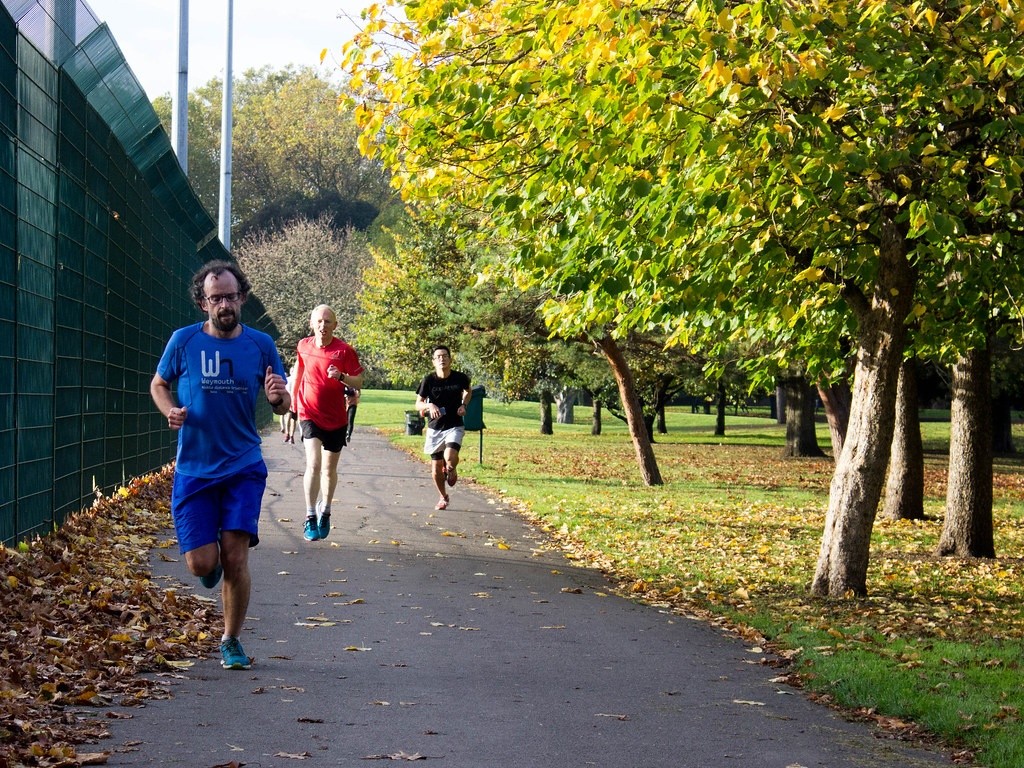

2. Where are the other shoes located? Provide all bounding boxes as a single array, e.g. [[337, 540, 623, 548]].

[[289, 438, 294, 443], [446, 464, 457, 486], [283, 435, 290, 442], [346, 435, 350, 442], [435, 494, 449, 510]]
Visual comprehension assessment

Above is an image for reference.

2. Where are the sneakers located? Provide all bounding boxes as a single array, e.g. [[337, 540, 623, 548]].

[[316, 500, 331, 539], [219, 636, 252, 670], [302, 514, 319, 541], [199, 528, 222, 588]]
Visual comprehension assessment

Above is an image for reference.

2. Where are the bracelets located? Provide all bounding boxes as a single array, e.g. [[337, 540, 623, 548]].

[[338, 373, 345, 382], [462, 404, 468, 411], [267, 398, 283, 407]]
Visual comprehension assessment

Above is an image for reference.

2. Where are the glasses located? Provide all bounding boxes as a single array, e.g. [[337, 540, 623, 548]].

[[433, 354, 450, 360], [203, 293, 240, 304]]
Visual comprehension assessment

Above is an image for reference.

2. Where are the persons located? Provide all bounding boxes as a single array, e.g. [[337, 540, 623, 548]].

[[415, 345, 471, 511], [345, 387, 361, 442], [280, 367, 303, 444], [151, 261, 291, 670], [290, 304, 364, 542]]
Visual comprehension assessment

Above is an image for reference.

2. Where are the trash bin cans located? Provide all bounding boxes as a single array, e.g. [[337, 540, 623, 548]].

[[404, 409, 423, 436], [462, 384, 486, 431]]
